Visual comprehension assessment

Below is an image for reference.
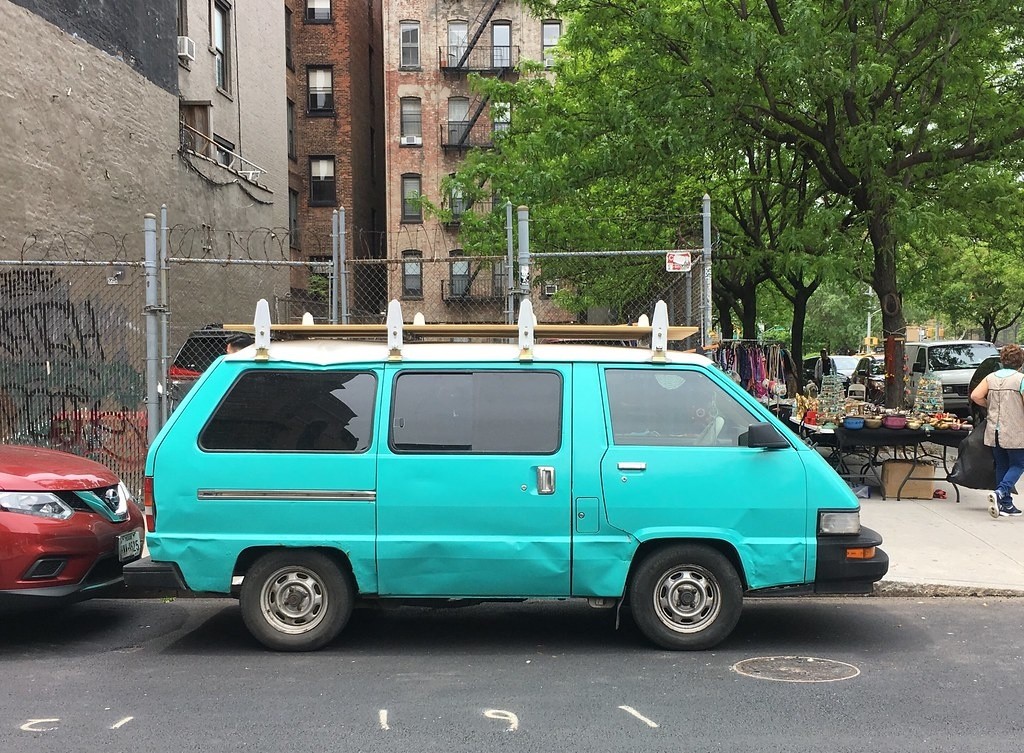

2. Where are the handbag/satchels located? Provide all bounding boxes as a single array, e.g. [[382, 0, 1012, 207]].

[[946, 418, 1018, 494]]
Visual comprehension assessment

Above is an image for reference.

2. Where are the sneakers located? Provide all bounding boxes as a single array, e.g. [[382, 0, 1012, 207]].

[[987, 490, 1003, 518], [999, 506, 1022, 516]]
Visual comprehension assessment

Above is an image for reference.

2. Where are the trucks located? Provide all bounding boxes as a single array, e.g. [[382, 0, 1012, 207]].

[[903, 340, 1001, 408]]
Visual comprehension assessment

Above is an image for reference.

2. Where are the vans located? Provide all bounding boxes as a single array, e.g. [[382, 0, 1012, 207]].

[[144, 344, 890, 652]]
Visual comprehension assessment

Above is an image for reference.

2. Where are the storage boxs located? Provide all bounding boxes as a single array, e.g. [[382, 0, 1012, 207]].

[[881, 459, 938, 500]]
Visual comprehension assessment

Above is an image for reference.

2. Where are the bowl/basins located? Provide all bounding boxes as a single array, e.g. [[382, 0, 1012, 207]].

[[865, 419, 882, 428], [843, 418, 864, 428], [907, 423, 921, 429], [883, 417, 906, 428]]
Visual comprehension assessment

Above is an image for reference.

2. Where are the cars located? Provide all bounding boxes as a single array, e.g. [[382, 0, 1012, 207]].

[[0, 445, 145, 609], [803, 355, 885, 405]]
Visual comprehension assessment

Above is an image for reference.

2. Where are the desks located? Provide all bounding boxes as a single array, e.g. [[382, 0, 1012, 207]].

[[769, 404, 973, 503]]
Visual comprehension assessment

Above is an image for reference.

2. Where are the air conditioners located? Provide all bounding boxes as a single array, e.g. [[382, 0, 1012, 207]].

[[401, 136, 422, 144], [545, 285, 557, 294], [178, 36, 195, 60]]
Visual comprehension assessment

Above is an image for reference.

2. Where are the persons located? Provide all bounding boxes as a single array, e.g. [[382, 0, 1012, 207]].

[[971, 344, 1024, 518], [815, 350, 837, 390]]
